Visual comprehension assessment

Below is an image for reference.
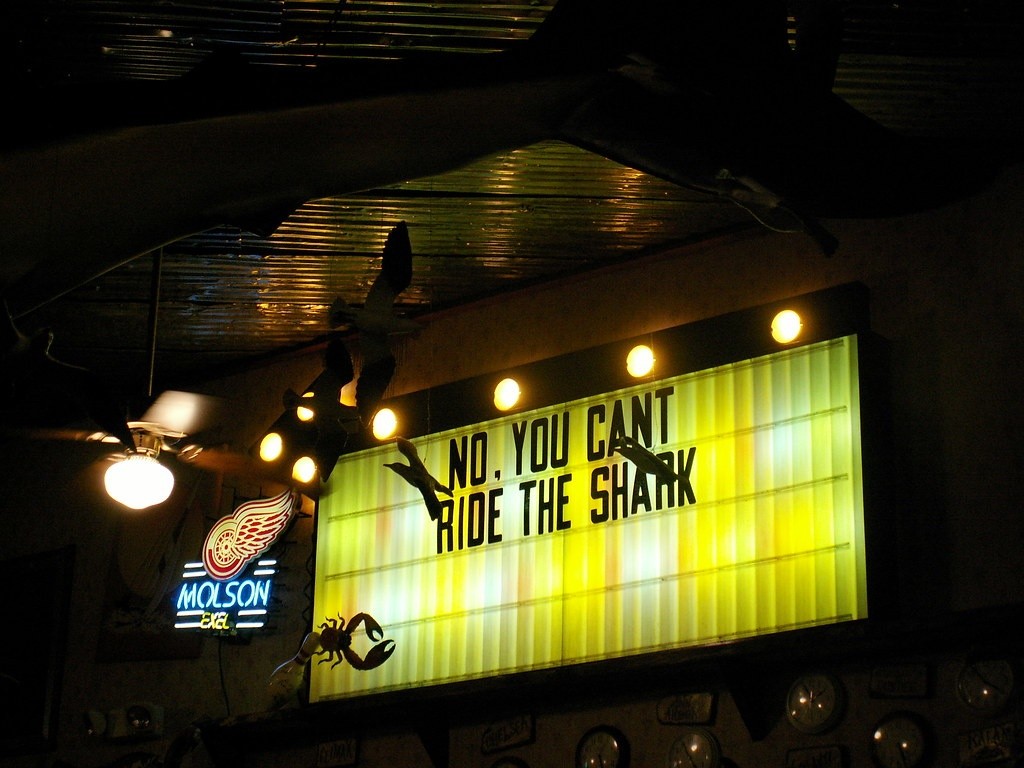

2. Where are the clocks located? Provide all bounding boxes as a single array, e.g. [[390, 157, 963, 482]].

[[666, 726, 719, 768], [571, 723, 631, 768], [784, 668, 846, 735], [866, 710, 938, 768], [957, 652, 1013, 718]]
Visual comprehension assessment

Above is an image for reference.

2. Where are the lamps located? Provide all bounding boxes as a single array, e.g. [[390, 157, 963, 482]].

[[104, 416, 174, 512]]
